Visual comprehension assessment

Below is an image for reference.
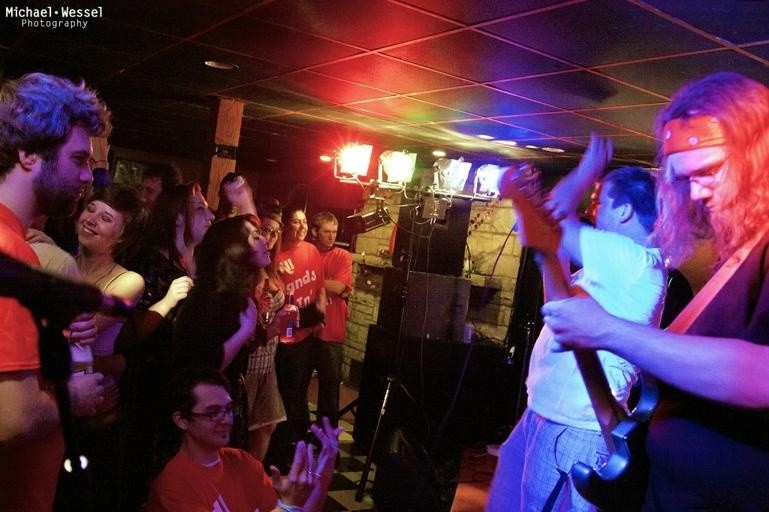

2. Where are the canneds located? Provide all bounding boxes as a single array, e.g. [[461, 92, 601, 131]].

[[281, 305, 300, 344]]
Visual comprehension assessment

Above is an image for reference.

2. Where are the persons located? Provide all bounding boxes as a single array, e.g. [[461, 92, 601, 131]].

[[473, 128, 667, 512], [540, 69, 769, 512], [0, 68, 358, 510]]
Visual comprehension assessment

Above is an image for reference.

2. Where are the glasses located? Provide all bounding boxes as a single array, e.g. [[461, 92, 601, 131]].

[[190, 408, 239, 421]]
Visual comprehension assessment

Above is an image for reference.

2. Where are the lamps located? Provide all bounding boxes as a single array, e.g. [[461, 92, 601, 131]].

[[433, 155, 473, 194], [379, 149, 418, 183], [333, 142, 374, 179], [473, 164, 502, 199]]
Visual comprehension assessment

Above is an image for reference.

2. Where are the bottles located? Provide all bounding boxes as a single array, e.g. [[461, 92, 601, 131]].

[[279, 290, 298, 345], [255, 278, 270, 335], [70, 340, 93, 375]]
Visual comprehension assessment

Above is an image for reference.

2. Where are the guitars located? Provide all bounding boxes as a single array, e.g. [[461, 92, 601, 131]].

[[499, 163, 667, 511]]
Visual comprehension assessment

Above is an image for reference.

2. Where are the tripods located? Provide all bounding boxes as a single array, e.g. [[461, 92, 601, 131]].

[[338, 298, 445, 504]]
